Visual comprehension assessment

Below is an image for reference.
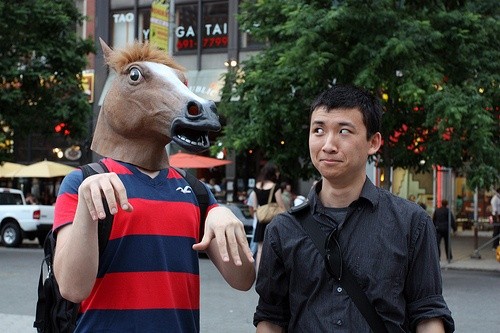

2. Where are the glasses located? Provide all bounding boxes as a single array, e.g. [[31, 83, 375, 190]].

[[320, 228, 343, 281]]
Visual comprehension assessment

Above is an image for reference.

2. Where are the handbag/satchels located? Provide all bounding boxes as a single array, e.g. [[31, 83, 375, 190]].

[[257, 183, 285, 225]]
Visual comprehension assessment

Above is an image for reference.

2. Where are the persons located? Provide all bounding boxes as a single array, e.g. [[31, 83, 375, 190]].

[[491, 189, 500, 249], [254, 87, 456, 333], [199, 177, 222, 196], [249, 161, 295, 281], [433, 199, 458, 263], [52, 38, 255, 333]]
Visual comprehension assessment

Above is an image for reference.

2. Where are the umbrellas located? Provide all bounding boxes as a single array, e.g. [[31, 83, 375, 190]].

[[0, 161, 80, 177], [169, 150, 231, 168]]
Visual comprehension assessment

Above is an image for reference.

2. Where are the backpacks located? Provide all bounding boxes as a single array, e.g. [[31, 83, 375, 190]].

[[33, 160, 209, 333]]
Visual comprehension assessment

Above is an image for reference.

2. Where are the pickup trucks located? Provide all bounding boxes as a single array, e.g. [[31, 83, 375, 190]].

[[0, 188, 56, 246]]
